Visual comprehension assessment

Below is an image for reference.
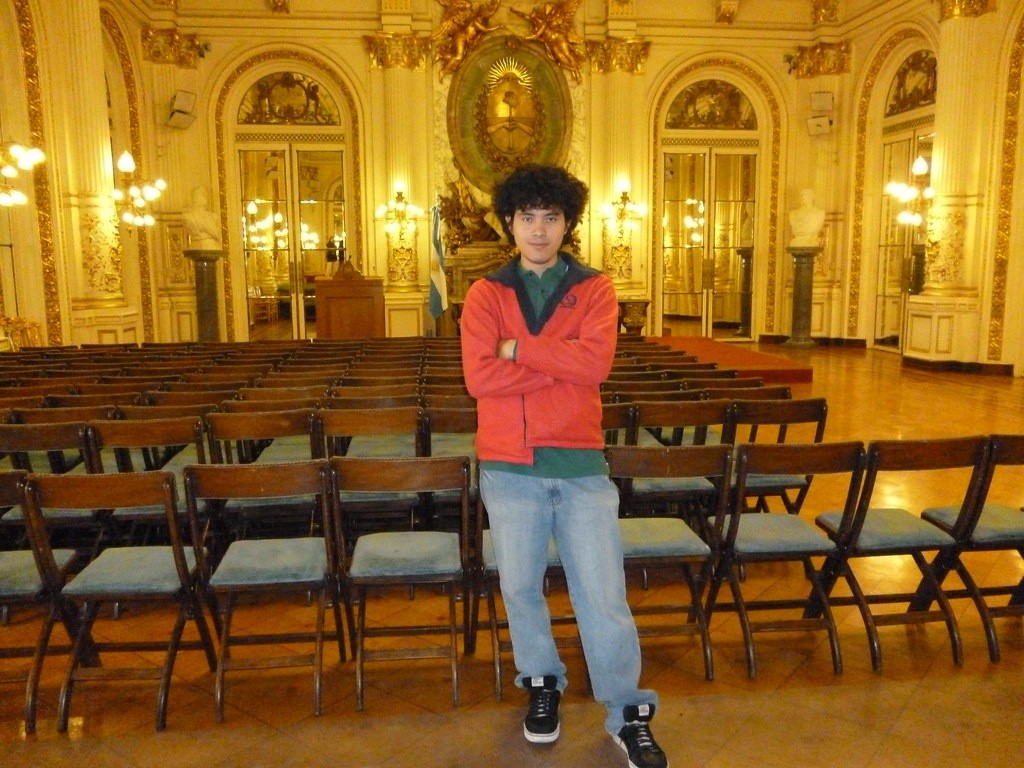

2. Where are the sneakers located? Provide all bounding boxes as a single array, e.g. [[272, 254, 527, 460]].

[[522, 674, 561, 743], [611, 704, 669, 768]]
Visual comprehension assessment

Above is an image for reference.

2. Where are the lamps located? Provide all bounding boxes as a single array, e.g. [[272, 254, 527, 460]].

[[597, 177, 649, 238], [884, 157, 938, 229], [109, 148, 168, 237], [372, 178, 431, 242], [0, 137, 45, 206]]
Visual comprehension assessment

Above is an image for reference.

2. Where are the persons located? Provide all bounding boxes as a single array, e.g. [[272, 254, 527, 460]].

[[509, 7, 581, 83], [461, 162, 668, 768], [789, 188, 825, 248], [439, 0, 506, 84], [182, 186, 221, 250], [326, 236, 344, 276]]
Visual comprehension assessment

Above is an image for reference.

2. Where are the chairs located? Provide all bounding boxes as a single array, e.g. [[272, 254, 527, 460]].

[[0, 335, 1021, 734]]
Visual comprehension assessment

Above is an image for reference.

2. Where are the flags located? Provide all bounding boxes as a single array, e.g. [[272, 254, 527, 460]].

[[429, 209, 448, 319]]
